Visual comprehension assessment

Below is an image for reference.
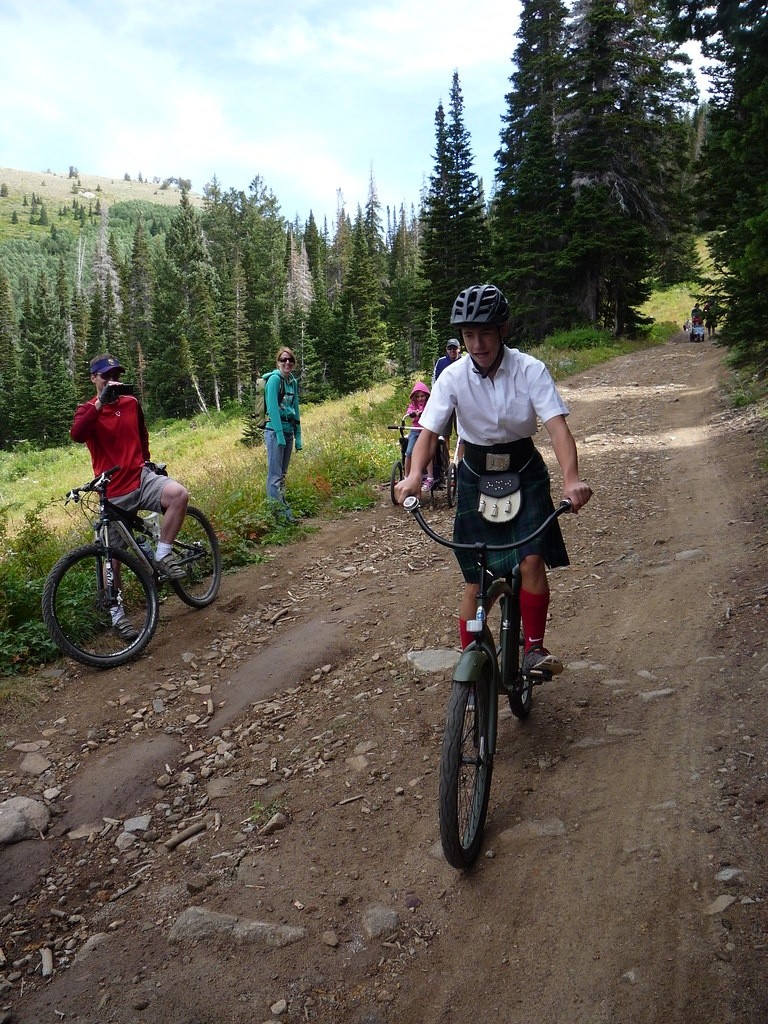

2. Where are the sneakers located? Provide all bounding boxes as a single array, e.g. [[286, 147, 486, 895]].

[[465, 688, 476, 711], [114, 616, 137, 639], [522, 646, 563, 676], [152, 554, 187, 579]]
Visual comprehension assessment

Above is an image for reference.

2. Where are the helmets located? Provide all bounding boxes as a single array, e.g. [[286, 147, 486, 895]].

[[450, 285, 509, 327]]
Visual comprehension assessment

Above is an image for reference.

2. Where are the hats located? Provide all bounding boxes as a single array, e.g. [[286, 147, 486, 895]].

[[447, 339, 460, 347], [90, 358, 125, 373]]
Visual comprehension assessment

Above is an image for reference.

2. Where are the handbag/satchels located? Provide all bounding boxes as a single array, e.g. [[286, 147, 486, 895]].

[[478, 473, 522, 524]]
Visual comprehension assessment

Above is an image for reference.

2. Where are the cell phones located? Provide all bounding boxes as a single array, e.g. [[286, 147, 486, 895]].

[[114, 384, 134, 395]]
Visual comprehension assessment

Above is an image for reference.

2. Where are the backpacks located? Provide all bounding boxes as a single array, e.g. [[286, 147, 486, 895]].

[[255, 376, 299, 429]]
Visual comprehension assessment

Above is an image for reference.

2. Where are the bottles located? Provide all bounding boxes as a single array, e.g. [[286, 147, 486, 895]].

[[136, 534, 156, 559]]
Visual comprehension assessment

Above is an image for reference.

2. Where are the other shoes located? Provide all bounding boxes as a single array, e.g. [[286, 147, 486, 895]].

[[421, 479, 434, 491]]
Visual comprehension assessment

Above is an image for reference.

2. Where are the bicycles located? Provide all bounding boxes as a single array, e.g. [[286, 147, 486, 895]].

[[393, 490, 575, 871], [39, 462, 225, 669]]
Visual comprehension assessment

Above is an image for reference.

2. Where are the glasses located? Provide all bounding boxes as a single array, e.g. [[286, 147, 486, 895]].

[[447, 346, 458, 350], [95, 370, 120, 380], [279, 357, 294, 362]]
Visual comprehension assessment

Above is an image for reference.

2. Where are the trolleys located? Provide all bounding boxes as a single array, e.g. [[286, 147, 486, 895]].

[[386, 411, 457, 509], [689, 317, 704, 343]]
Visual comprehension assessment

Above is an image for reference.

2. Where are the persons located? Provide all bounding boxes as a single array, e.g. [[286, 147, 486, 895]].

[[703, 298, 719, 339], [404, 381, 436, 493], [690, 302, 704, 328], [432, 336, 464, 476], [262, 348, 303, 526], [392, 285, 593, 681], [68, 353, 190, 642]]
